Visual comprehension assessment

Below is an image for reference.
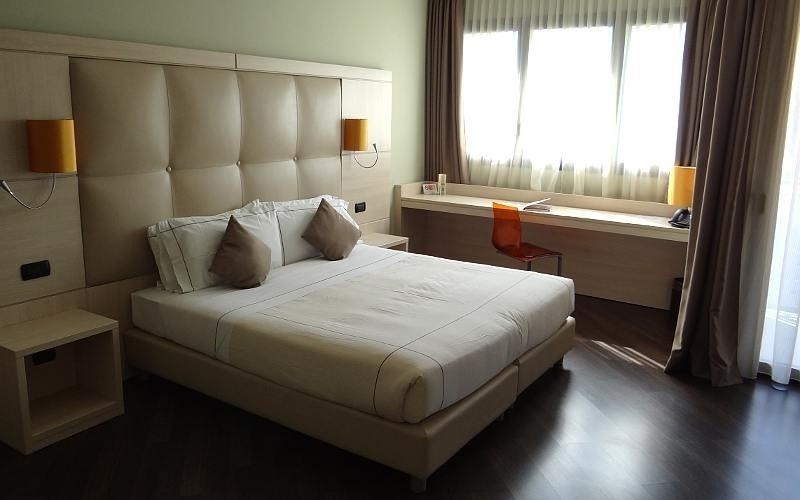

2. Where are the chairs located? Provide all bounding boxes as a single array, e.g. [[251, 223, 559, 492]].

[[492, 202, 563, 277]]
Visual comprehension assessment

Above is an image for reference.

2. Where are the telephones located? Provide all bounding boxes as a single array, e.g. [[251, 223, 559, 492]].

[[668, 208, 690, 228]]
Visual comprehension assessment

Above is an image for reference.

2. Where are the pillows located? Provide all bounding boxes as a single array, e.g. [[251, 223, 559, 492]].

[[147, 198, 261, 294], [276, 200, 349, 266], [207, 214, 272, 288], [156, 202, 283, 295], [301, 198, 363, 263], [310, 194, 349, 209]]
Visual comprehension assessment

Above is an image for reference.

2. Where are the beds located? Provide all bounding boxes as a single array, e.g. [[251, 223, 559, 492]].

[[124, 248, 575, 490]]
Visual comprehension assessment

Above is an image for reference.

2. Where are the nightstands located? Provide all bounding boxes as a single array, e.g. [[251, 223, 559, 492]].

[[0, 308, 125, 458], [361, 232, 409, 251]]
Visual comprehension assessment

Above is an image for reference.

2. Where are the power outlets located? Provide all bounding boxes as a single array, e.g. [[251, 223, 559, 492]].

[[20, 260, 50, 280], [355, 203, 366, 213]]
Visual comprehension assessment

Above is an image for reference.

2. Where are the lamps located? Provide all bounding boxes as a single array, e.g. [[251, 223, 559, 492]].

[[0, 119, 77, 209], [668, 165, 699, 227], [344, 118, 378, 168]]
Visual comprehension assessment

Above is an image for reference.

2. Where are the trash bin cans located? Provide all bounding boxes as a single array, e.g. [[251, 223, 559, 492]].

[[670, 278, 683, 322]]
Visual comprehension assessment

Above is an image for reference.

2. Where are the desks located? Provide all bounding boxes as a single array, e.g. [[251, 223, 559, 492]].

[[393, 180, 692, 311]]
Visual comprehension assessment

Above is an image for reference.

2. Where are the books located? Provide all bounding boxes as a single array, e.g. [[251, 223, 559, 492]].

[[523, 197, 553, 213]]
